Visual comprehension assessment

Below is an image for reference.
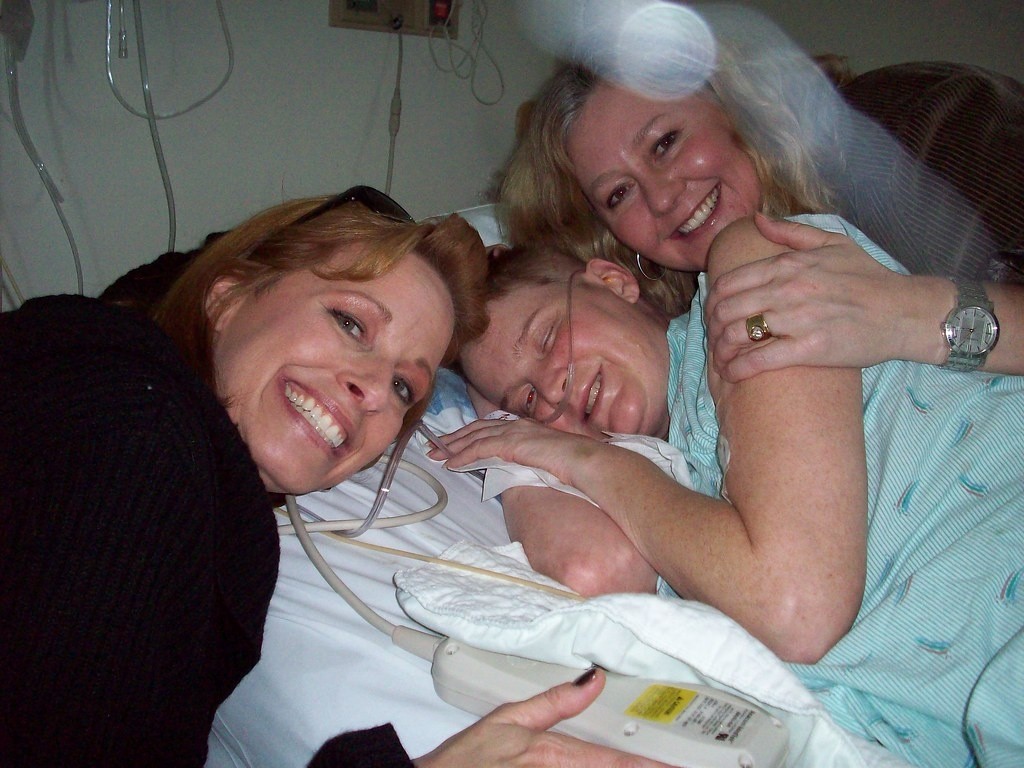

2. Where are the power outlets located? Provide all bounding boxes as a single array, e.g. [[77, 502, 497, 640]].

[[328, 0, 459, 41]]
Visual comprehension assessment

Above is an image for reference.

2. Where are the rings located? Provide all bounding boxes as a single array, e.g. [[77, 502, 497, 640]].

[[746, 312, 772, 342]]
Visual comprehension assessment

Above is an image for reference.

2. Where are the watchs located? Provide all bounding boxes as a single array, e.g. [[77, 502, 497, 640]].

[[939, 274, 1001, 373]]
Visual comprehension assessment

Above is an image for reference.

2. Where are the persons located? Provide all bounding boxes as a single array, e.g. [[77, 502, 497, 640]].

[[0, 185, 676, 768], [429, 212, 1024, 768], [494, 30, 1024, 384]]
